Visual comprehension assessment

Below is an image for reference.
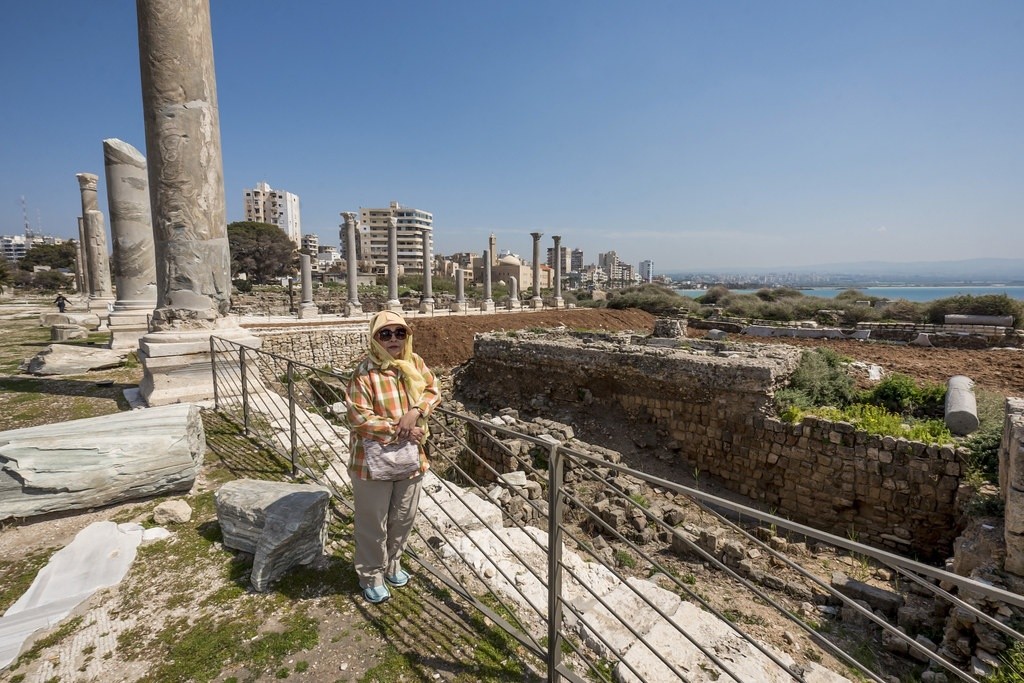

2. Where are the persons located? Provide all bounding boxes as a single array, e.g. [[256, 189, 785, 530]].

[[345, 310, 443, 605], [53, 291, 74, 313]]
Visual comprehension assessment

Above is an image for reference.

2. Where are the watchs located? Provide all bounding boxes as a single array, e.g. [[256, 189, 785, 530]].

[[413, 407, 422, 417]]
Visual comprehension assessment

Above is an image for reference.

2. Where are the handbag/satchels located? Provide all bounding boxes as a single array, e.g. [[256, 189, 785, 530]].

[[363, 435, 421, 481]]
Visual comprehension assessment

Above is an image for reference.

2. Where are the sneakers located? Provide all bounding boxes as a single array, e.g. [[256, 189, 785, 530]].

[[384, 569, 411, 587], [358, 581, 390, 603]]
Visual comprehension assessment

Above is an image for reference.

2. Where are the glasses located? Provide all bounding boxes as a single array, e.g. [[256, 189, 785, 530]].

[[379, 327, 407, 341]]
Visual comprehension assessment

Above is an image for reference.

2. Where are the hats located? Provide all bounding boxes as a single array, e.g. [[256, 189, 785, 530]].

[[372, 312, 413, 338]]
